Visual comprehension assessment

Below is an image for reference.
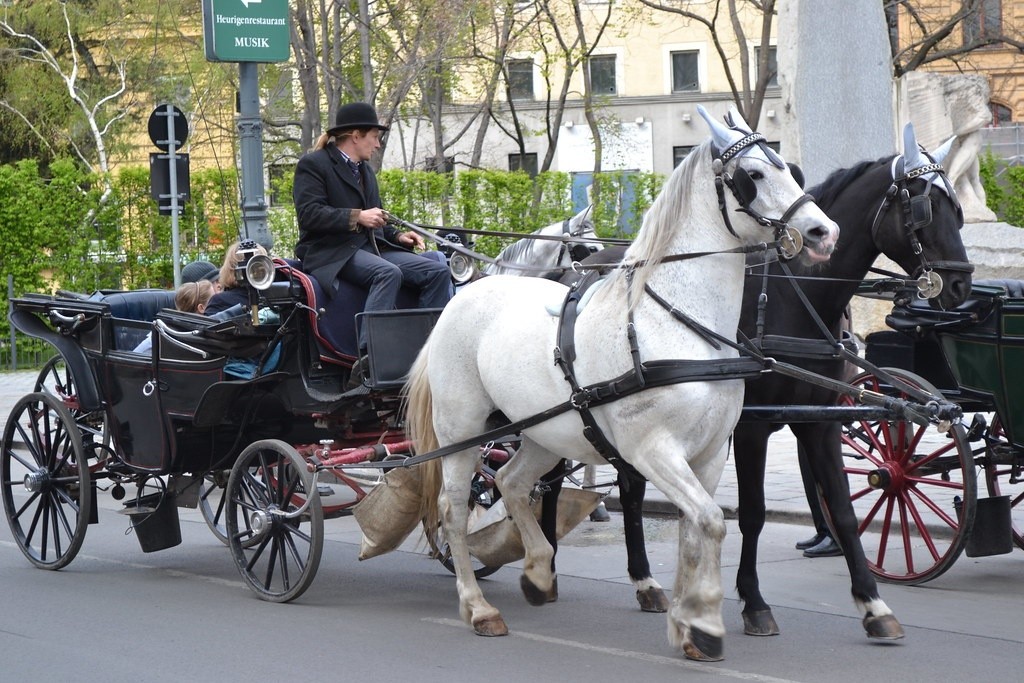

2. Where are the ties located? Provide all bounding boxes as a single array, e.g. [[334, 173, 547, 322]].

[[347, 160, 363, 184]]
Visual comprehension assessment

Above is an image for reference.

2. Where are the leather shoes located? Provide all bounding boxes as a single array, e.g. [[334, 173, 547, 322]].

[[803, 536, 843, 558], [795, 533, 822, 550]]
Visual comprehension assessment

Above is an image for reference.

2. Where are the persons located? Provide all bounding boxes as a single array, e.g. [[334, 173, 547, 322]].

[[787, 163, 857, 556], [293, 102, 452, 390], [181, 260, 222, 294], [132, 279, 214, 356], [205, 241, 272, 367], [429, 227, 490, 557]]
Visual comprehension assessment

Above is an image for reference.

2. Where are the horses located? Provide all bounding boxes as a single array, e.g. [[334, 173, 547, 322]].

[[403, 104, 977, 663]]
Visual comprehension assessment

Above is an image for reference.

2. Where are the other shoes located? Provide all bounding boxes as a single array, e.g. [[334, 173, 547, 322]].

[[345, 355, 369, 391]]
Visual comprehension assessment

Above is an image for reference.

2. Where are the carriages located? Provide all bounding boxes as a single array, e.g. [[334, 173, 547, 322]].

[[0, 103, 976, 661]]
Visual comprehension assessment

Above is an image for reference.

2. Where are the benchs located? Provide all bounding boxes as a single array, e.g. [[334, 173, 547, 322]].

[[80, 289, 176, 355], [273, 242, 452, 381], [158, 275, 299, 377], [885, 272, 1024, 464]]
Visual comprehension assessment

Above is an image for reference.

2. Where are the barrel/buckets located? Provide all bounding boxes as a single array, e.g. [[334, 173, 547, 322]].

[[952, 495, 1013, 558], [122, 475, 183, 553]]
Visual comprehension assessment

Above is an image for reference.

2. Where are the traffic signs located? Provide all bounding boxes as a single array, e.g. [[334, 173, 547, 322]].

[[211, 0, 290, 62]]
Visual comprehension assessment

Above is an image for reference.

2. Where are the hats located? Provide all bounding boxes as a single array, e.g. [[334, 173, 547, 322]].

[[326, 103, 389, 134], [181, 261, 221, 284], [435, 224, 475, 258]]
